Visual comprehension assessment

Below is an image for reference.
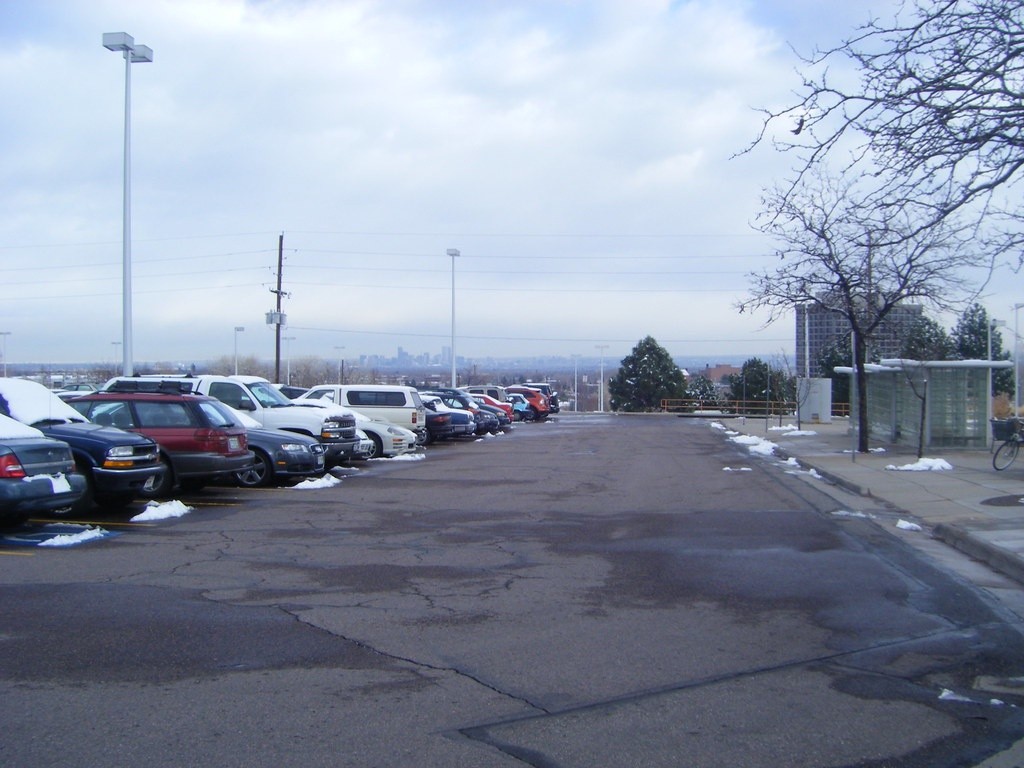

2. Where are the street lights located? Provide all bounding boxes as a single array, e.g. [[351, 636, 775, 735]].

[[103, 32, 154, 380], [446, 249, 460, 386]]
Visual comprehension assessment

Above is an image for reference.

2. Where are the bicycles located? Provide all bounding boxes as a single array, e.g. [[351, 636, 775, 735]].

[[989, 416, 1024, 471]]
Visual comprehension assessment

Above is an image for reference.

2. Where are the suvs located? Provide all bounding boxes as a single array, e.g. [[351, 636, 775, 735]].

[[419, 388, 500, 437], [521, 383, 560, 417], [102, 372, 361, 480], [61, 390, 255, 498], [504, 386, 551, 421], [0, 377, 168, 520]]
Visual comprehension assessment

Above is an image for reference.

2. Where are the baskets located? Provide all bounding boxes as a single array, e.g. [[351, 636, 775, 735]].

[[990, 418, 1019, 442]]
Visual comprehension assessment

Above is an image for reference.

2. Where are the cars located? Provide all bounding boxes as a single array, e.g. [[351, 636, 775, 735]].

[[1, 412, 89, 532], [272, 382, 420, 458], [472, 398, 510, 426], [418, 395, 476, 442], [505, 393, 532, 421], [412, 405, 452, 447], [223, 402, 324, 487]]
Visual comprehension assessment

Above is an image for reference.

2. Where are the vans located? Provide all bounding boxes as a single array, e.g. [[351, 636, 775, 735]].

[[456, 385, 508, 402], [294, 384, 427, 445]]
